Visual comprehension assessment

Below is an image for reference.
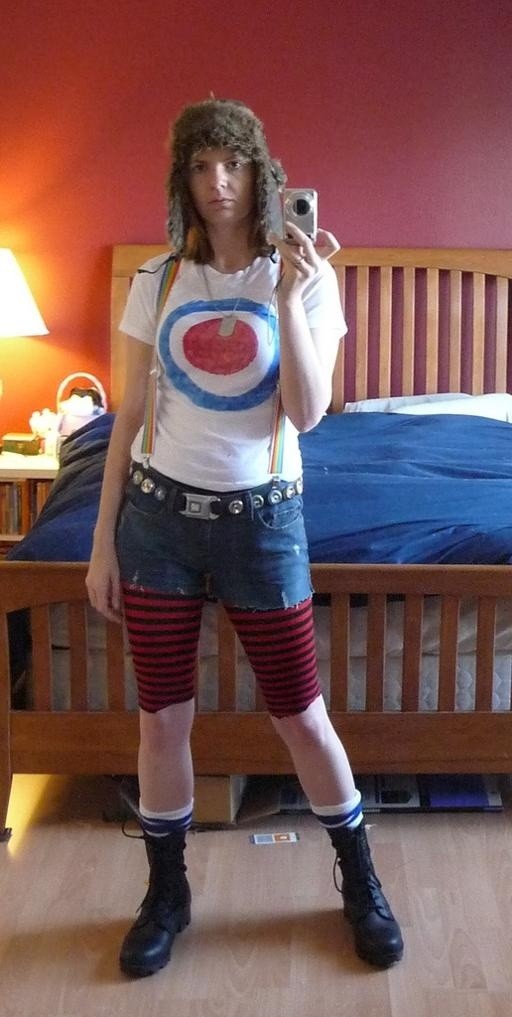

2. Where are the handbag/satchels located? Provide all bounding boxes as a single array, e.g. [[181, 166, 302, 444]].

[[55, 371, 107, 438]]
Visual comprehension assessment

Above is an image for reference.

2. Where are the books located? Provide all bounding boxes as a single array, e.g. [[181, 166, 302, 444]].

[[0, 478, 53, 560]]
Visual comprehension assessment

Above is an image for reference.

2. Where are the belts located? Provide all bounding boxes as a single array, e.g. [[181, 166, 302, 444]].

[[126, 460, 303, 521]]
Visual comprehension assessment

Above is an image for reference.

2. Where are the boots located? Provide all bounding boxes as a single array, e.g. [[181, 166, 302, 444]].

[[326, 817, 404, 967], [120, 827, 192, 978]]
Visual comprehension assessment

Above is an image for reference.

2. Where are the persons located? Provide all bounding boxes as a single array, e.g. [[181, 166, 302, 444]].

[[80, 90, 408, 982]]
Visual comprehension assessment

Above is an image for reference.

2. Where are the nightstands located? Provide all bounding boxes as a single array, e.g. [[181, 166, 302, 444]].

[[0, 449, 62, 558]]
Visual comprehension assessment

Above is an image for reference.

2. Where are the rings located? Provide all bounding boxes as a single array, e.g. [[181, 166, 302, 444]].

[[295, 257, 305, 267]]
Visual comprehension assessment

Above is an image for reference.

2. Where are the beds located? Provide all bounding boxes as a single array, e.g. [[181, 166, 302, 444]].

[[0, 241, 511, 840]]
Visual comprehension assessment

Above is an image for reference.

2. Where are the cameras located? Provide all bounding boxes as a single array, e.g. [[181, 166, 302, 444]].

[[282, 188, 318, 246]]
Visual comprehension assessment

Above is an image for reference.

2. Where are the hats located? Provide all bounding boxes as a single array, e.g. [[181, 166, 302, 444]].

[[164, 97, 286, 258]]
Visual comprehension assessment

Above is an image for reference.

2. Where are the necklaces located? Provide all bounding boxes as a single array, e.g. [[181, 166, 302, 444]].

[[200, 254, 256, 338]]
